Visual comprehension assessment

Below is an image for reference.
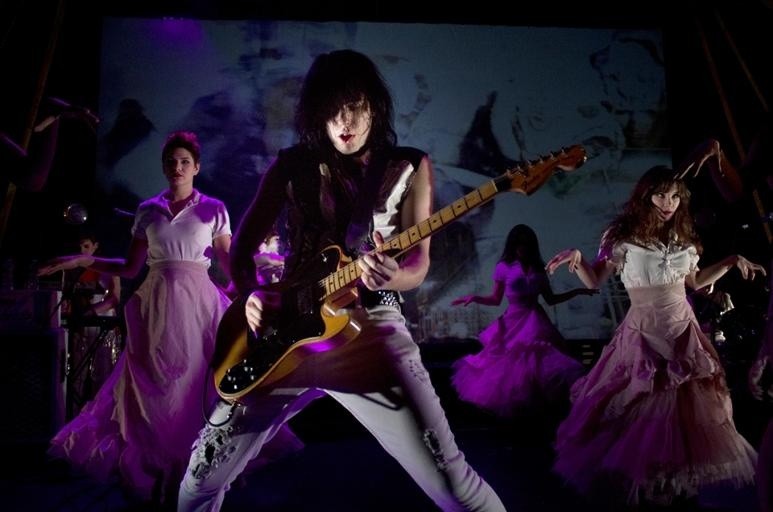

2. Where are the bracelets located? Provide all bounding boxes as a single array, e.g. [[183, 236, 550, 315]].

[[91, 308, 97, 315]]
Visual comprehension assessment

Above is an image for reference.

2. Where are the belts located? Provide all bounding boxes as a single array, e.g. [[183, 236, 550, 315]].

[[345, 289, 403, 309]]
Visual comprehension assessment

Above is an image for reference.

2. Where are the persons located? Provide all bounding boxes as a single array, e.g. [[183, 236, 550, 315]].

[[39, 230, 120, 423], [39, 132, 231, 512], [544, 165, 767, 500], [219, 229, 286, 300], [178, 49, 506, 512], [449, 224, 600, 412]]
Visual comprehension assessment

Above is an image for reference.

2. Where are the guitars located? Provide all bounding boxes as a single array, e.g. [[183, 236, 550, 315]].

[[214, 144, 586, 407]]
